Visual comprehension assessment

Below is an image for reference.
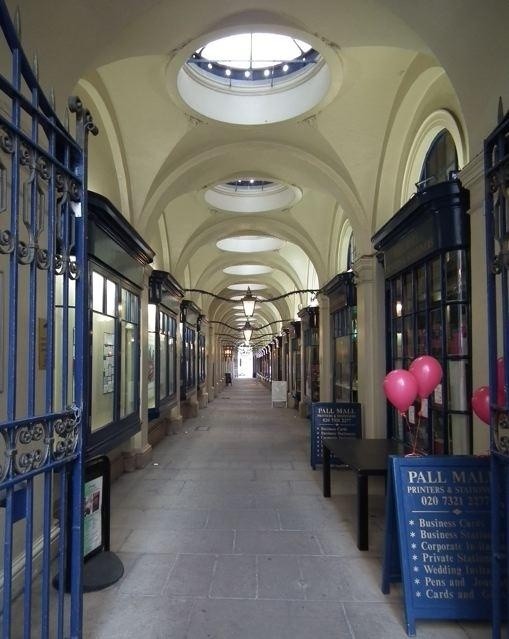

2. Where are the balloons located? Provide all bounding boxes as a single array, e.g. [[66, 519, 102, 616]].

[[471, 385, 491, 424], [383, 368, 419, 416], [497, 357, 505, 407], [408, 355, 444, 400]]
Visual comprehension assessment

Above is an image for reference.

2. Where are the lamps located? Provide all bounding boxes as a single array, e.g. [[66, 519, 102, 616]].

[[207, 318, 291, 341], [185, 285, 319, 317]]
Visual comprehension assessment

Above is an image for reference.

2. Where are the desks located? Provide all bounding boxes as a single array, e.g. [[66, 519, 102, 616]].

[[322, 439, 427, 551]]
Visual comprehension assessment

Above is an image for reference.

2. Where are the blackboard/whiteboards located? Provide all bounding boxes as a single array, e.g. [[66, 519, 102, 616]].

[[271, 380, 288, 402], [392, 455, 509, 621], [310, 402, 362, 464]]
